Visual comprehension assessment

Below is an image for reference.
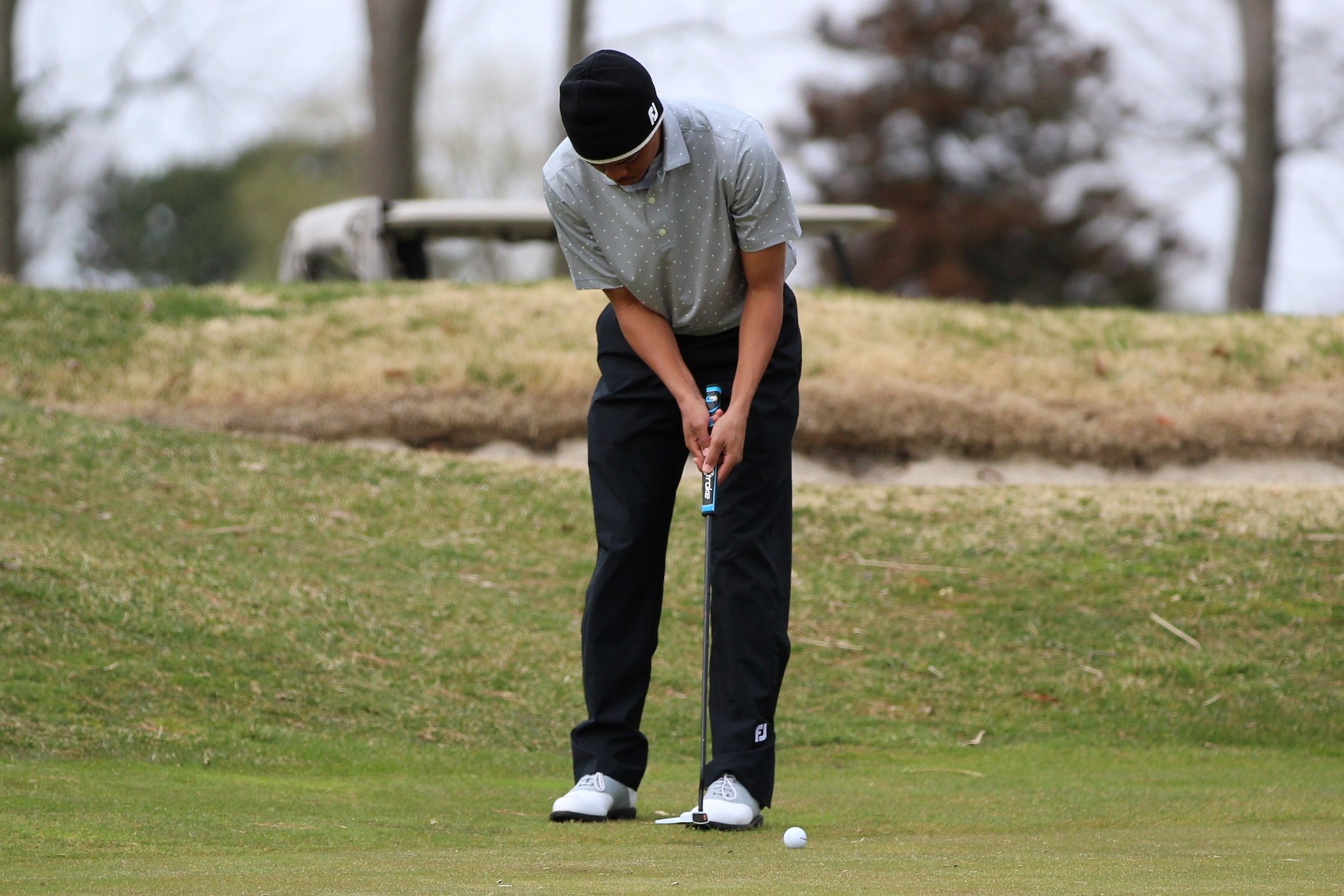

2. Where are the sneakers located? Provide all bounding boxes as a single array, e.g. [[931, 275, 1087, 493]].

[[550, 770, 638, 819], [689, 775, 763, 830]]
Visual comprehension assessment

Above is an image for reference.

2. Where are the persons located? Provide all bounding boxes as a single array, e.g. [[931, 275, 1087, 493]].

[[540, 48, 802, 829]]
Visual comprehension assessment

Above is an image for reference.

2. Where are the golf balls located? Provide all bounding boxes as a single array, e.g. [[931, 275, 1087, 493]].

[[783, 827, 807, 849]]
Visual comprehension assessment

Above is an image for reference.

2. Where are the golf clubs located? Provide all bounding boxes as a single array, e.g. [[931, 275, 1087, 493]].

[[654, 385, 724, 826]]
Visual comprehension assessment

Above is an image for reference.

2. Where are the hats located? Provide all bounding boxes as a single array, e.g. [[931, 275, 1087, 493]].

[[559, 49, 666, 162]]
